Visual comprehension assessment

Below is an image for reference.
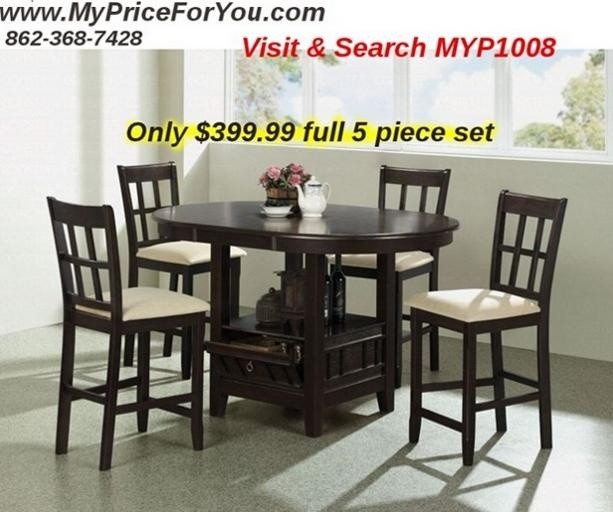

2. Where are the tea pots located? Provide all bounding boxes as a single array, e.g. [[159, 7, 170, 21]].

[[295, 175, 331, 218], [254, 286, 280, 326]]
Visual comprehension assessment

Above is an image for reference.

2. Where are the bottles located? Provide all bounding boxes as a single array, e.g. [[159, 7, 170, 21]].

[[324, 253, 347, 324]]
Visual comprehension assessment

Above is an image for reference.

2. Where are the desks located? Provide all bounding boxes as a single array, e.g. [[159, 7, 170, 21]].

[[153, 201, 458, 439]]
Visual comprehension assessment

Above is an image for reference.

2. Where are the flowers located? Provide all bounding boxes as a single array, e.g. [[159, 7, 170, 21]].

[[258, 161, 311, 192]]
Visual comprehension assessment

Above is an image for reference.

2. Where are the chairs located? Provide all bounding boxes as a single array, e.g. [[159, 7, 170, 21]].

[[44, 160, 246, 472], [323, 165, 568, 465]]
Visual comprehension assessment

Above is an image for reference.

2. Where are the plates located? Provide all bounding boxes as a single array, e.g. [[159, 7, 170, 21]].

[[260, 211, 294, 218]]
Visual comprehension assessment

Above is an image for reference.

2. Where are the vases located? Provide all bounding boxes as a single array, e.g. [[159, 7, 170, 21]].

[[265, 186, 297, 212]]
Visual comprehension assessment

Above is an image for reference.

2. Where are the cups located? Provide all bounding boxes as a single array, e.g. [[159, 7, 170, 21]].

[[261, 205, 292, 212]]
